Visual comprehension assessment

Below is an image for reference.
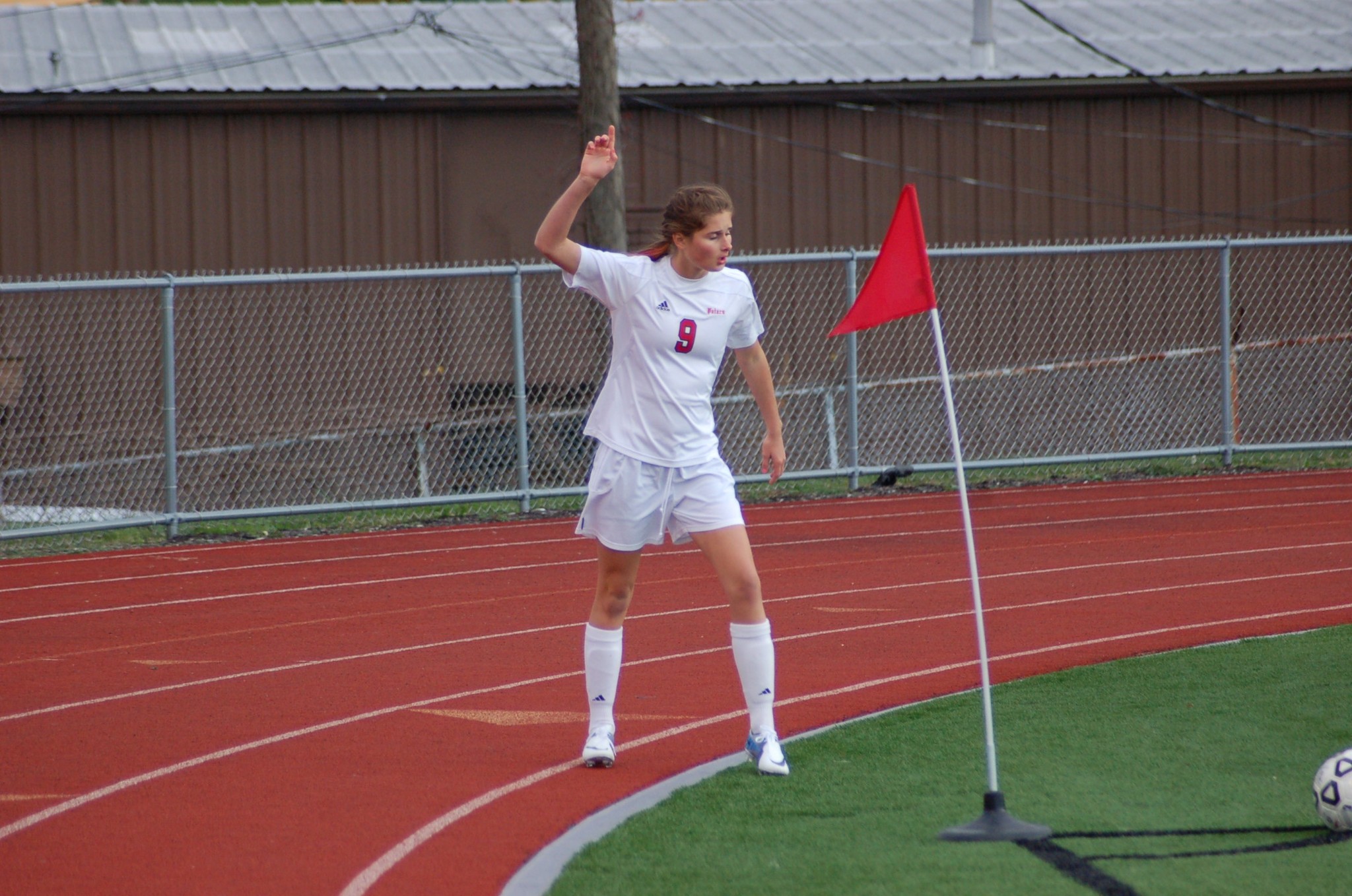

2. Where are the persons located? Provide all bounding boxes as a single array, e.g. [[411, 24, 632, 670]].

[[532, 123, 791, 777]]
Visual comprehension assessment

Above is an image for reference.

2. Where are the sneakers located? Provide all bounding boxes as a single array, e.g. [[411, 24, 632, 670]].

[[746, 727, 790, 775], [583, 732, 616, 766]]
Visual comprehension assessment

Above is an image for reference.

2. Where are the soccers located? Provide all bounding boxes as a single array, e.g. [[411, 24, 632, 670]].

[[1311, 749, 1352, 833]]
